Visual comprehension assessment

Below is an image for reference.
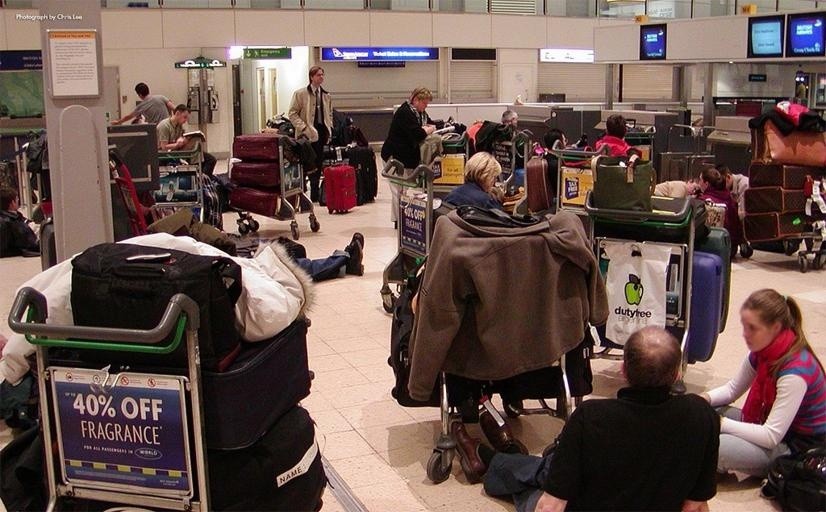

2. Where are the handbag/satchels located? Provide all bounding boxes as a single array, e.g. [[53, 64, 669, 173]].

[[345, 125, 369, 147], [591, 155, 653, 223], [71, 242, 241, 330]]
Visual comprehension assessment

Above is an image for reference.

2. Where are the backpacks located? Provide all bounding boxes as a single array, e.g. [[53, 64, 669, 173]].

[[761, 449, 826, 511]]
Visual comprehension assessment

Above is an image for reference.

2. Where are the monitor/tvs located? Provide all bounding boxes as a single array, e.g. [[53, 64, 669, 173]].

[[786, 11, 826, 57], [640, 23, 666, 60], [747, 14, 785, 58]]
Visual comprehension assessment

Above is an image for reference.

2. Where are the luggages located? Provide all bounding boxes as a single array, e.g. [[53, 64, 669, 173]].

[[349, 147, 378, 205], [589, 196, 732, 361], [229, 135, 301, 219], [44, 404, 334, 512], [325, 163, 356, 213], [388, 297, 592, 407], [47, 319, 316, 450], [742, 166, 826, 242]]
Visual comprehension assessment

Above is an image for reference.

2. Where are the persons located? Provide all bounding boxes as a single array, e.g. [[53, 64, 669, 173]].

[[501, 110, 518, 131], [288, 66, 334, 205], [449, 324, 719, 512], [156, 104, 229, 213], [170, 221, 365, 283], [380, 85, 450, 229], [542, 114, 752, 264], [689, 288, 826, 483], [0, 188, 41, 257], [443, 151, 504, 211], [111, 83, 175, 126]]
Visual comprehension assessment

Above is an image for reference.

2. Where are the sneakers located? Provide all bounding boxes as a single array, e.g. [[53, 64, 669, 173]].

[[346, 234, 365, 276]]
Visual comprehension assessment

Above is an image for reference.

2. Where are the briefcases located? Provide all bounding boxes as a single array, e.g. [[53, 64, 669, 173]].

[[526, 156, 553, 212], [743, 112, 826, 168]]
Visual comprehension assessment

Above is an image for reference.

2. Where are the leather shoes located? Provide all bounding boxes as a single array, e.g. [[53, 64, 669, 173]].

[[480, 410, 529, 455], [451, 421, 485, 483]]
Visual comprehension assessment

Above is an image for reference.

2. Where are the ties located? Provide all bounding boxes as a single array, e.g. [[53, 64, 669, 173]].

[[315, 88, 322, 123]]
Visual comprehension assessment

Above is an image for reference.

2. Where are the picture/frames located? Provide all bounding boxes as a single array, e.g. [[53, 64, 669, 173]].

[[48, 27, 103, 101]]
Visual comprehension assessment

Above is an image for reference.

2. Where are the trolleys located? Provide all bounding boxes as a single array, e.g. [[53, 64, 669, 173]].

[[4, 285, 212, 512], [410, 204, 587, 486], [228, 135, 321, 240], [154, 131, 224, 233], [104, 148, 153, 240], [379, 123, 826, 397]]
